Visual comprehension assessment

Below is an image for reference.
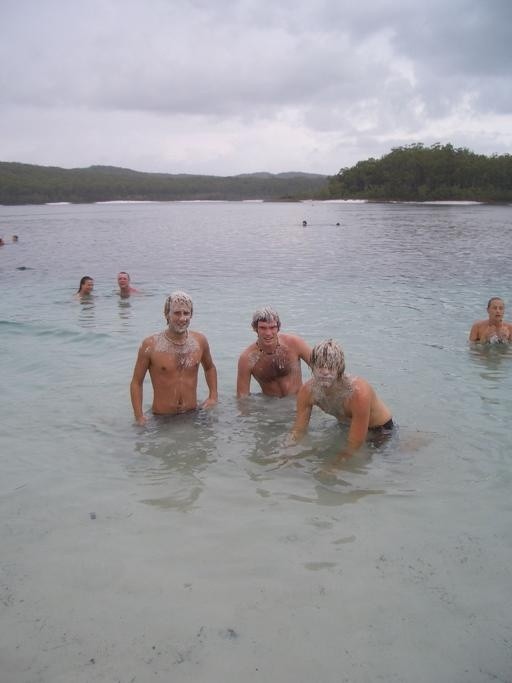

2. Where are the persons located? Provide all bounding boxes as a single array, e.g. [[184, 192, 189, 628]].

[[127, 290, 220, 429], [234, 307, 315, 411], [75, 276, 94, 295], [116, 271, 137, 298], [468, 296, 511, 347], [285, 338, 397, 465], [13, 235, 19, 241], [1, 239, 6, 245]]
